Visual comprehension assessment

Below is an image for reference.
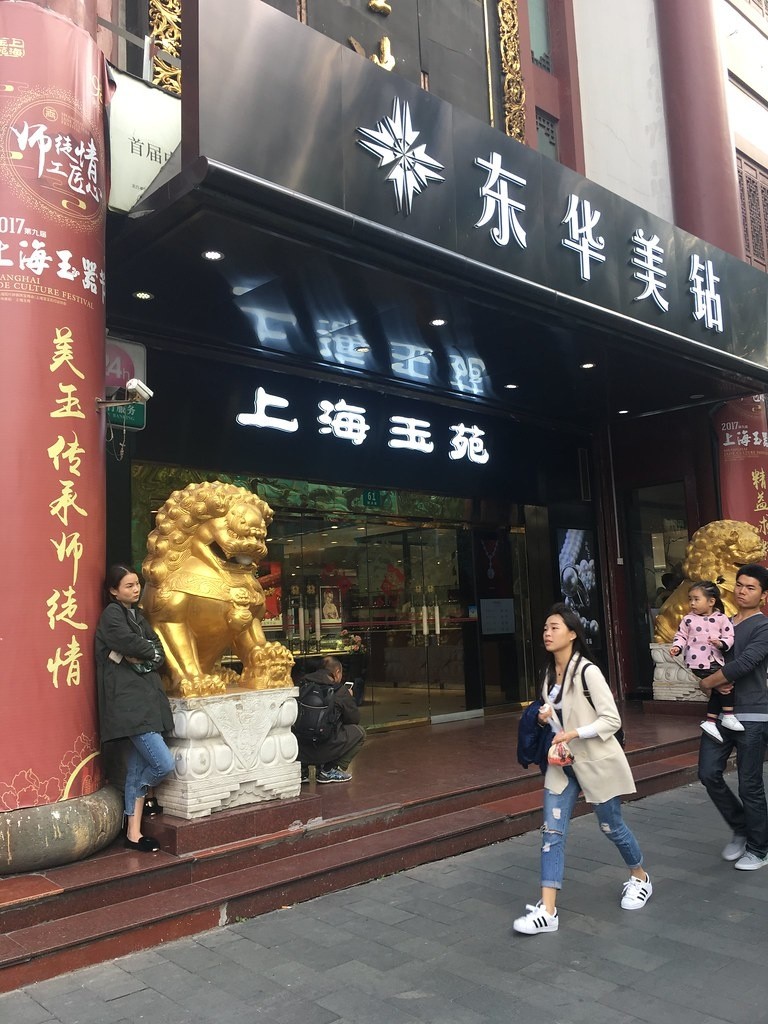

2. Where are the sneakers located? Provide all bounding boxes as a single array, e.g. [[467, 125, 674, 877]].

[[721, 715, 745, 731], [513, 899, 558, 934], [699, 721, 723, 744], [735, 850, 768, 870], [317, 768, 352, 783], [301, 767, 310, 783], [720, 835, 748, 860], [621, 872, 653, 910]]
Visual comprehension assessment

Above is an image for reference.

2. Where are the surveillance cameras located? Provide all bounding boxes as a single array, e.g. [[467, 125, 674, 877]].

[[126, 378, 154, 403]]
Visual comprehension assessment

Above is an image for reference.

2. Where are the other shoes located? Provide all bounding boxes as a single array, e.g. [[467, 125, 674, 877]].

[[125, 836, 160, 851], [143, 797, 163, 815]]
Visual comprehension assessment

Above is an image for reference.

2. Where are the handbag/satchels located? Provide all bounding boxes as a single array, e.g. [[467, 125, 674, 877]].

[[546, 704, 576, 766]]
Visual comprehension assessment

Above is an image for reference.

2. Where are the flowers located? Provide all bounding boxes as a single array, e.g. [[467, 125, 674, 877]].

[[340, 629, 370, 657]]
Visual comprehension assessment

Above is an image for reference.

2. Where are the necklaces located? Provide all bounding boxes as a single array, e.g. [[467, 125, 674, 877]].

[[481, 536, 499, 579], [559, 529, 600, 633]]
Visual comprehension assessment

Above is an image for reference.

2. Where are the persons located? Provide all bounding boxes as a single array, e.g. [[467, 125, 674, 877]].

[[669, 581, 745, 743], [294, 656, 367, 783], [514, 607, 652, 935], [323, 589, 339, 619], [699, 564, 768, 870], [95, 566, 176, 852]]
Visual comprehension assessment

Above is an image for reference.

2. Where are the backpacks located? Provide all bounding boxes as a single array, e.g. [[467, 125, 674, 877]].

[[291, 676, 343, 745], [582, 663, 625, 750]]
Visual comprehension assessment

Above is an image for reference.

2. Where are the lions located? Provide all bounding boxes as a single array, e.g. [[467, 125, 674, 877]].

[[652, 519, 767, 643], [135, 479, 295, 695]]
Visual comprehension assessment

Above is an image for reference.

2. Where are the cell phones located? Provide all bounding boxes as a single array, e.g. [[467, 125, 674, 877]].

[[343, 682, 354, 690], [108, 651, 124, 665]]
